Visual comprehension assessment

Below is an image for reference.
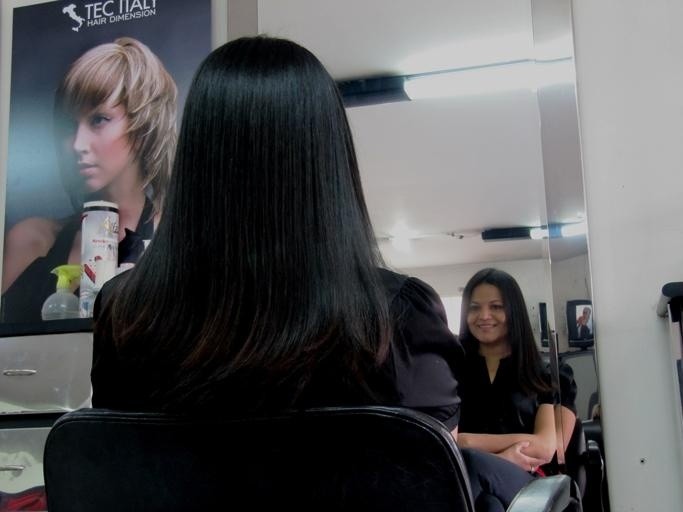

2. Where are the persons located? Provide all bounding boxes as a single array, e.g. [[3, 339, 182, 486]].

[[459, 269, 577, 512], [1, 37, 179, 336], [93, 37, 458, 432]]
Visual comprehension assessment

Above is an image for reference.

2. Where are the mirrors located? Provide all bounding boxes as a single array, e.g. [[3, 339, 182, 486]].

[[226, 0, 610, 512]]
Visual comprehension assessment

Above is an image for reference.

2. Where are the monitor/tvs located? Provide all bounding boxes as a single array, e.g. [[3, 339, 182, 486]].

[[566, 299, 594, 351]]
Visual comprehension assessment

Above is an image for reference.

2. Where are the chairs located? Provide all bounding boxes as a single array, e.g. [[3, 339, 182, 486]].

[[41, 403, 585, 512]]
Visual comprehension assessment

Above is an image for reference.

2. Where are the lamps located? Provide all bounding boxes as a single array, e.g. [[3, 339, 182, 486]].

[[339, 59, 538, 108]]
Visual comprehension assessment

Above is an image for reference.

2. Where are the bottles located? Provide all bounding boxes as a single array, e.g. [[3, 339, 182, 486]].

[[79, 201, 119, 321]]
